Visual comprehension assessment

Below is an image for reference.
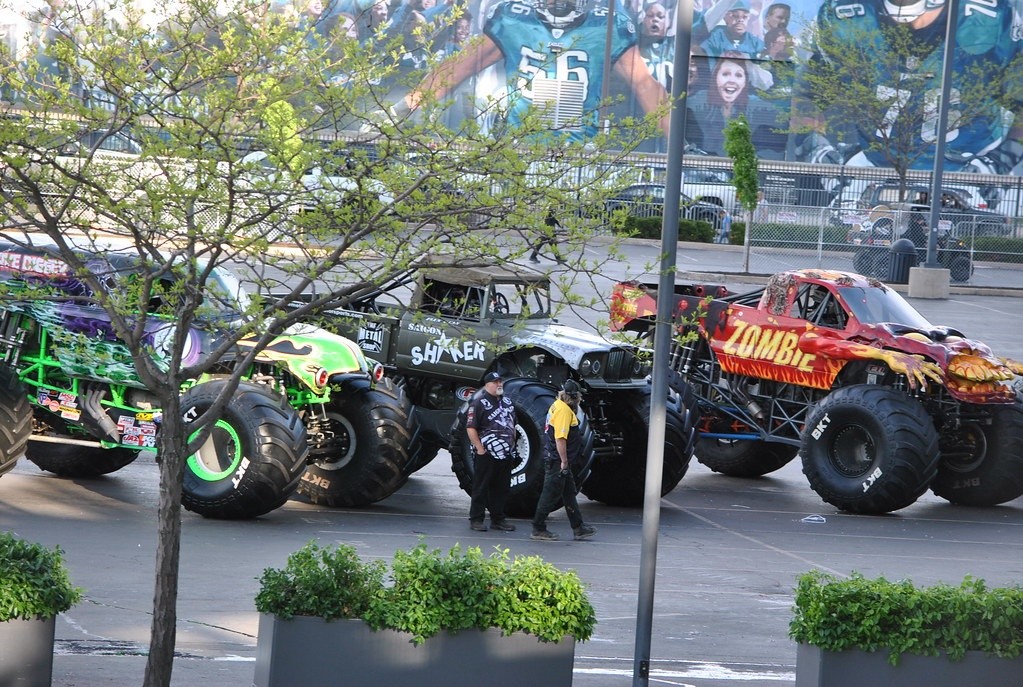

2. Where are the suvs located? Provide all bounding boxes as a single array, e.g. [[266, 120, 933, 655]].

[[0, 235, 422, 522], [853, 178, 1013, 242]]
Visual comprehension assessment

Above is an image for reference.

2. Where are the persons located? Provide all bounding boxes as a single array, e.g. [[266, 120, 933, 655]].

[[529, 378, 600, 541], [943, 194, 948, 206], [918, 192, 928, 204], [529, 205, 568, 265], [465, 371, 517, 531], [719, 209, 732, 243], [755, 190, 769, 225]]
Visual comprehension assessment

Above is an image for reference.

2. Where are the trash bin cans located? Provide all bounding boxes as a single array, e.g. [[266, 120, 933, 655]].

[[886, 238, 919, 284]]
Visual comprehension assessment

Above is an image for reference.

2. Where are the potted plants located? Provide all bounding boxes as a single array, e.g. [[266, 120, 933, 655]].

[[787, 568, 1023, 687], [0, 529, 88, 687], [254, 534, 598, 687]]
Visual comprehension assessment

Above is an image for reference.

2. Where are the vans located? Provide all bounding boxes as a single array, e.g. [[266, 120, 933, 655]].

[[593, 159, 743, 217]]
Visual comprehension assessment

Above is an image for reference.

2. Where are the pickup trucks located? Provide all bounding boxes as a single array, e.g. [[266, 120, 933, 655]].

[[242, 253, 697, 521], [608, 267, 1023, 516]]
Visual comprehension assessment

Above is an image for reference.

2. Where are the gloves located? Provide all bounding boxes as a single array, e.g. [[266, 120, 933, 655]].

[[360, 106, 399, 132], [946, 149, 1010, 209], [559, 465, 573, 479], [794, 133, 845, 195]]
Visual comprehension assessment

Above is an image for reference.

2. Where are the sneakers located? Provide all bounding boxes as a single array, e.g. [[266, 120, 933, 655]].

[[530, 527, 558, 540], [573, 522, 597, 540], [471, 520, 488, 531], [490, 519, 516, 530]]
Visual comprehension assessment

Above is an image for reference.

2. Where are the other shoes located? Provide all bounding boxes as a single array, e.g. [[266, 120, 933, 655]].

[[529, 257, 540, 263], [558, 259, 567, 265]]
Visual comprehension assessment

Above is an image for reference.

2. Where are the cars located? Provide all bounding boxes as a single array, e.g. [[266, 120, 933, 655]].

[[391, 177, 502, 229], [579, 182, 730, 240], [828, 192, 862, 231], [234, 145, 396, 219], [0, 109, 145, 157]]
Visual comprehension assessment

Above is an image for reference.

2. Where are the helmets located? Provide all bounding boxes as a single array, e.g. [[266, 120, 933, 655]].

[[531, 0, 587, 28]]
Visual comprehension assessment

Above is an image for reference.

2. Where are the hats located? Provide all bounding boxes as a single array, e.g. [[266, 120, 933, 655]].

[[729, 0, 750, 13], [484, 372, 503, 383], [565, 379, 587, 393]]
